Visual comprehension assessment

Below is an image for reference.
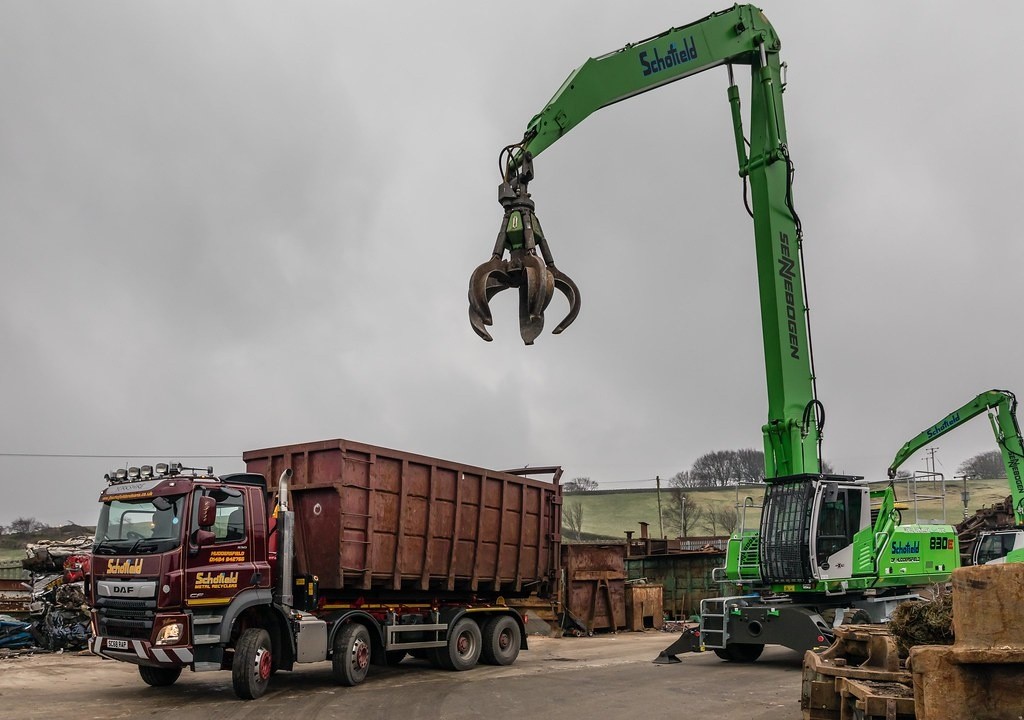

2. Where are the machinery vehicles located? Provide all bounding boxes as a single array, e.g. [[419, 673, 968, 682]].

[[467, 2, 1023, 665]]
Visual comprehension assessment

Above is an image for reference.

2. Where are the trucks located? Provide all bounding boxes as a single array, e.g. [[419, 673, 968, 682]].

[[85, 438, 566, 702]]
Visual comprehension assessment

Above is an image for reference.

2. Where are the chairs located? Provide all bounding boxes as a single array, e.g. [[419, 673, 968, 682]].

[[226, 509, 245, 540]]
